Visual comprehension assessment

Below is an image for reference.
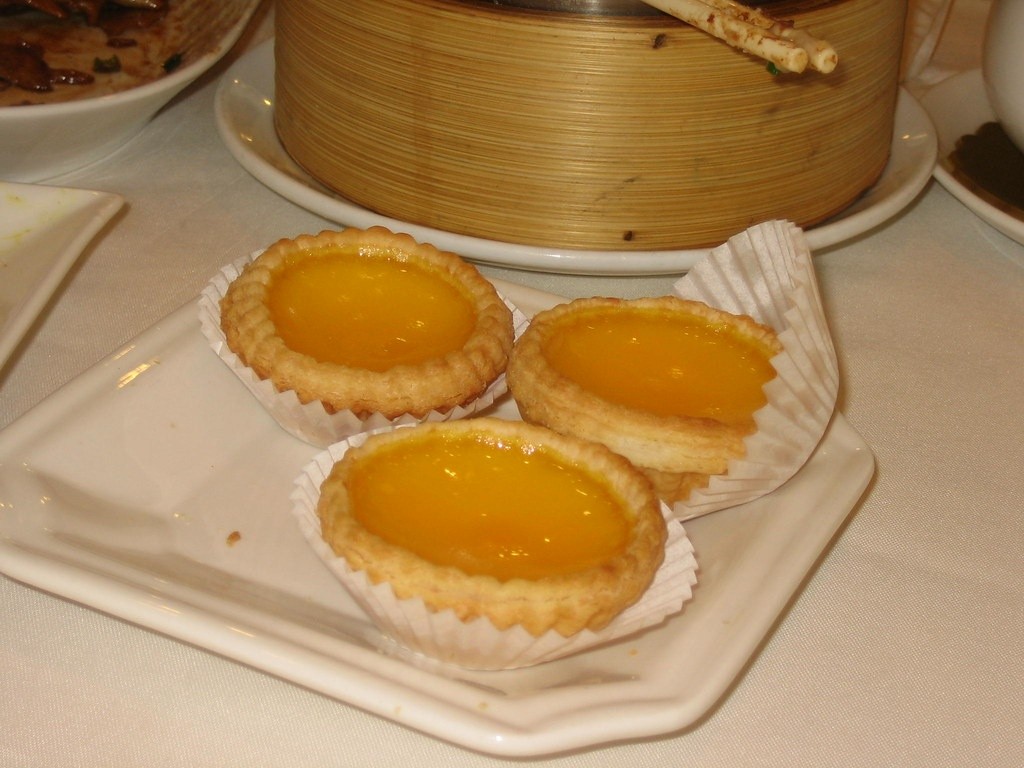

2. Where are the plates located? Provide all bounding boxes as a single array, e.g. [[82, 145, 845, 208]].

[[920, 69, 1024, 245], [218, 37, 939, 272], [0, 275, 875, 758], [0, 182, 125, 370]]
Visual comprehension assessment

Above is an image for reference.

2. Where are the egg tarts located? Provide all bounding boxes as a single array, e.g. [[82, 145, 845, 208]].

[[313, 416, 669, 637], [504, 296, 787, 515], [193, 222, 533, 449]]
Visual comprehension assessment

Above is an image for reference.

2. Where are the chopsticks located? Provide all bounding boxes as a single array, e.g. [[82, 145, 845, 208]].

[[643, 0, 838, 75]]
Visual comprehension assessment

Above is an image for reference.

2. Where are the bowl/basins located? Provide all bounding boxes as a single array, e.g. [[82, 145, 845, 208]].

[[0, 0, 260, 185]]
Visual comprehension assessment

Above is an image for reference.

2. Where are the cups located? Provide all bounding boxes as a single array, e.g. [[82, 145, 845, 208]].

[[983, 3, 1024, 147]]
